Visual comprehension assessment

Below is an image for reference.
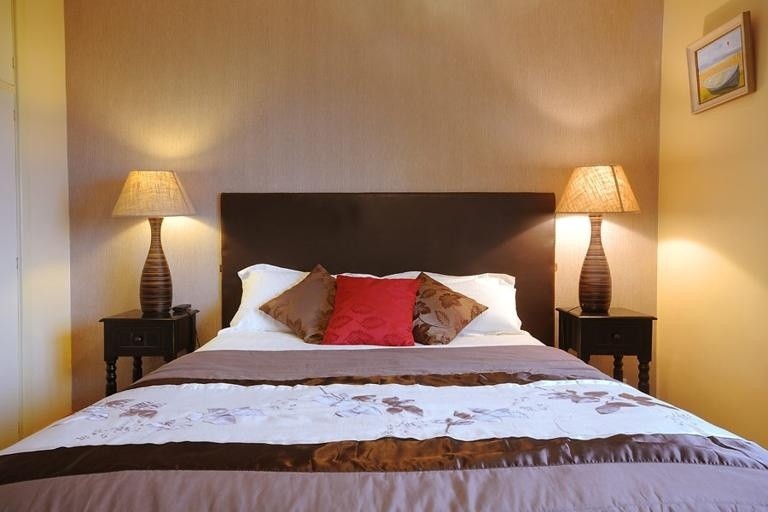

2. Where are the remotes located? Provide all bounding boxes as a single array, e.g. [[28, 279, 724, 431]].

[[173, 303, 191, 311]]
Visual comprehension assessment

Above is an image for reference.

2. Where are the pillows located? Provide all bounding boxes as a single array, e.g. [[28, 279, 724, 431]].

[[388, 268, 521, 334], [230, 264, 378, 328], [258, 263, 336, 345], [323, 274, 419, 346], [412, 270, 489, 345]]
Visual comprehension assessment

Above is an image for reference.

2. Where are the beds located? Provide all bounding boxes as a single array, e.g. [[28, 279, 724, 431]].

[[0, 327, 768, 511]]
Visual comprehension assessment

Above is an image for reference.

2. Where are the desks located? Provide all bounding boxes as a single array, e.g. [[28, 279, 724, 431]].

[[556, 305, 655, 393], [100, 307, 201, 397]]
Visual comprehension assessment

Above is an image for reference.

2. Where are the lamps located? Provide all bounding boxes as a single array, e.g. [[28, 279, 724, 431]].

[[552, 162, 639, 311], [112, 168, 194, 315]]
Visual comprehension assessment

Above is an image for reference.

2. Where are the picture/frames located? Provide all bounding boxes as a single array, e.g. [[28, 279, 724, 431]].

[[684, 11, 755, 114]]
